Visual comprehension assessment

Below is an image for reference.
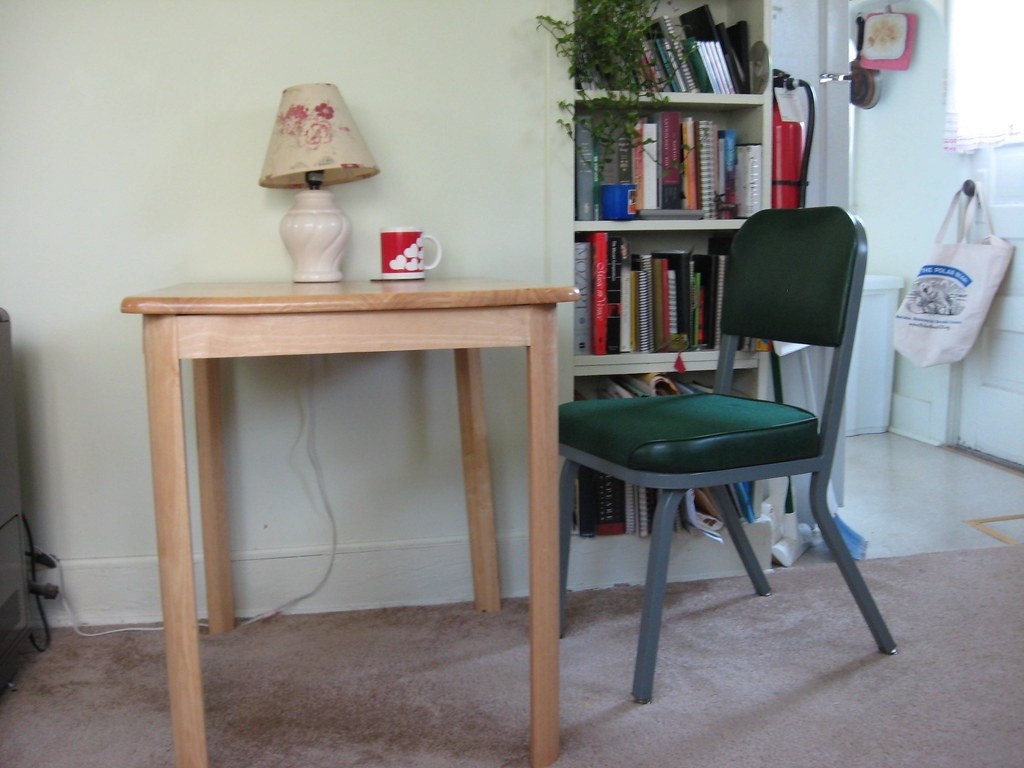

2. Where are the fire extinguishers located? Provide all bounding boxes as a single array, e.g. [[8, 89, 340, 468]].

[[771, 68, 817, 211]]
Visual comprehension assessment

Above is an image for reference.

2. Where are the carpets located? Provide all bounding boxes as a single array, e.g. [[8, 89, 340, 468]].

[[0, 542, 1024, 768]]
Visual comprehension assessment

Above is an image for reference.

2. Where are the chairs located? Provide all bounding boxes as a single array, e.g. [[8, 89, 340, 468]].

[[556, 206, 897, 706]]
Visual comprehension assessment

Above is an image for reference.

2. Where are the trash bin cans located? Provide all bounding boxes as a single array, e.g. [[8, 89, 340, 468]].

[[768, 276, 904, 436]]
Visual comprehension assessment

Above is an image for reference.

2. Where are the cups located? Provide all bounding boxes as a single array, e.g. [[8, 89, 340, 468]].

[[381, 231, 441, 280]]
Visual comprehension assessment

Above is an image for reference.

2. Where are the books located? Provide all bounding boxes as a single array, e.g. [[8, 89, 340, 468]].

[[572, 373, 756, 544], [574, 233, 772, 355], [576, 111, 762, 221], [578, 4, 750, 95]]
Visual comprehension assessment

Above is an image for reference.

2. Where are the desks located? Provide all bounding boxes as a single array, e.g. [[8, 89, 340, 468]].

[[121, 281, 581, 768]]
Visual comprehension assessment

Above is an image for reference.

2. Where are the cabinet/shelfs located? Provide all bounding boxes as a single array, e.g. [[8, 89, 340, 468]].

[[546, 1, 774, 590]]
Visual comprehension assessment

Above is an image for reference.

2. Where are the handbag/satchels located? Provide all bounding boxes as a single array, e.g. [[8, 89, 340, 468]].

[[893, 182, 1013, 368]]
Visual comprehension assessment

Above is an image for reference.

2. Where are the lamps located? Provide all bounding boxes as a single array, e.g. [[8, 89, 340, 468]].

[[258, 82, 383, 283]]
